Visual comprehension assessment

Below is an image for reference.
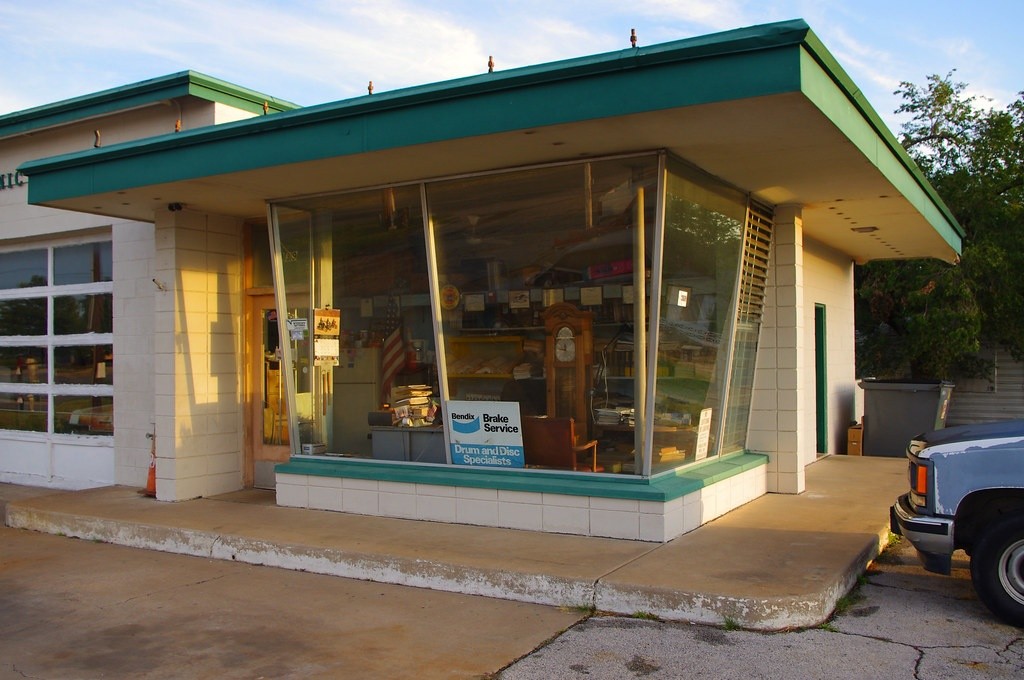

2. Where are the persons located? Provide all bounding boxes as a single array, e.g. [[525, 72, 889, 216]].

[[16, 353, 37, 383], [17, 393, 34, 411]]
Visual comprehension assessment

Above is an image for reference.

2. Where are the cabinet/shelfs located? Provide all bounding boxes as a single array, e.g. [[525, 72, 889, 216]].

[[369, 275, 720, 473]]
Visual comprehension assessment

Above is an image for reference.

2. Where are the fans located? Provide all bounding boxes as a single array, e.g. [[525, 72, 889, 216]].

[[442, 215, 514, 247]]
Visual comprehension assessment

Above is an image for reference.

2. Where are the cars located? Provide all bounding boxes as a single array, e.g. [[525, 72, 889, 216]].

[[53, 346, 94, 366], [890, 418, 1024, 626], [525, 248, 620, 288]]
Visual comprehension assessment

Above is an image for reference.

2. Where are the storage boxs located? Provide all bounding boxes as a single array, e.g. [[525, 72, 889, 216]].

[[848, 416, 865, 455], [587, 260, 633, 279]]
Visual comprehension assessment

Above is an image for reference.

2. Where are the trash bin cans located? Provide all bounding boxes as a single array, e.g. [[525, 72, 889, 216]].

[[859, 378, 955, 458]]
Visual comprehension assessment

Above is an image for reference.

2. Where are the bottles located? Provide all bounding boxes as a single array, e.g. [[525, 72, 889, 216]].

[[360, 329, 369, 342], [414, 348, 422, 361]]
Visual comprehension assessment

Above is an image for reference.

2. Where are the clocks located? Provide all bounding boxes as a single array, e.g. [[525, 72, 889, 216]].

[[542, 302, 593, 438]]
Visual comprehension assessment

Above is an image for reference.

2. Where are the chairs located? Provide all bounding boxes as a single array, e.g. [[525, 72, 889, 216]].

[[520, 414, 605, 474]]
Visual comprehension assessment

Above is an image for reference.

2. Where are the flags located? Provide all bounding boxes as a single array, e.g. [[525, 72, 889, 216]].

[[382, 288, 417, 400]]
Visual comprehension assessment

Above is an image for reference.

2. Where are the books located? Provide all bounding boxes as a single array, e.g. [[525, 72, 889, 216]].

[[595, 407, 634, 425], [583, 294, 716, 377]]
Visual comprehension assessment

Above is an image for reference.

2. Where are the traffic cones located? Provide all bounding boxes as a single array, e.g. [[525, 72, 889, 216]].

[[137, 427, 157, 497]]
